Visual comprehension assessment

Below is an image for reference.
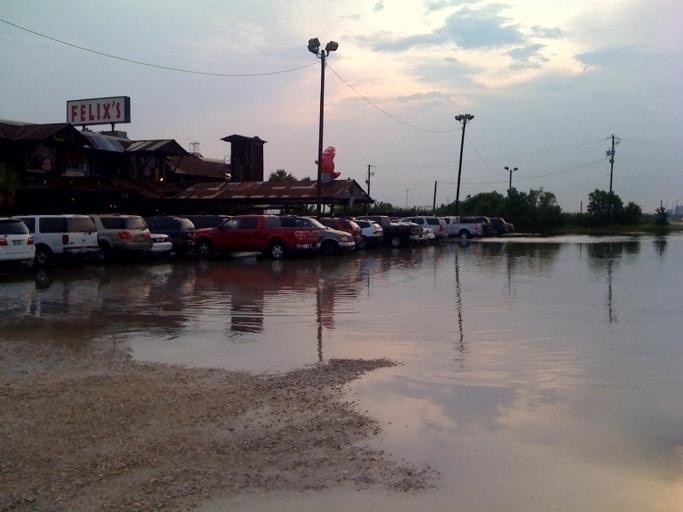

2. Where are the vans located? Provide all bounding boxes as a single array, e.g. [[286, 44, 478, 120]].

[[145, 215, 196, 255], [10, 214, 101, 269], [89, 214, 153, 259]]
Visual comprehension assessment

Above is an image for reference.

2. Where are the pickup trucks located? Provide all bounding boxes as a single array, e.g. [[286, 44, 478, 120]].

[[192, 214, 321, 261]]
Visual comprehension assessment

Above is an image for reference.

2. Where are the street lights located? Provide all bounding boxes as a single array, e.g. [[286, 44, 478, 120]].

[[307, 37, 339, 223], [504, 167, 518, 190], [454, 114, 474, 217]]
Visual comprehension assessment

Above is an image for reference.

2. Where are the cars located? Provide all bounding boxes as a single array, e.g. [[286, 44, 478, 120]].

[[351, 215, 515, 248], [278, 216, 356, 258], [149, 233, 173, 251]]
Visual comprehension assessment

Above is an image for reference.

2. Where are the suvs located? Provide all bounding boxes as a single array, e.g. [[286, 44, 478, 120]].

[[0, 217, 37, 281]]
